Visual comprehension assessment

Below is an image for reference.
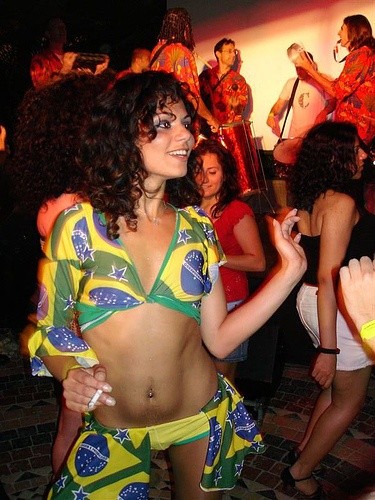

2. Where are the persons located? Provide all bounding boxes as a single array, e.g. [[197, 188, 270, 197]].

[[198, 38, 250, 123], [149, 8, 218, 139], [191, 140, 266, 390], [28, 69, 307, 499], [340, 256, 375, 359], [281, 121, 375, 500], [30, 18, 150, 88], [11, 72, 114, 475], [268, 14, 375, 146]]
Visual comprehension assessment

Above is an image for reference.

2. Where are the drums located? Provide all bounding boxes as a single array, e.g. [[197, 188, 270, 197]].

[[219, 121, 264, 197], [273, 136, 303, 178]]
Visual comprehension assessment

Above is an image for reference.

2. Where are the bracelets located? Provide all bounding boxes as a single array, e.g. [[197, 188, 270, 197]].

[[360, 319, 375, 341], [317, 346, 340, 354]]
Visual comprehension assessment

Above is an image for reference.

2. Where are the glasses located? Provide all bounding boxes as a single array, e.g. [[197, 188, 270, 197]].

[[219, 49, 239, 56]]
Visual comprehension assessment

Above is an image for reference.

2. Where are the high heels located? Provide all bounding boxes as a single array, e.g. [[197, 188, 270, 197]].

[[282, 466, 327, 500], [286, 446, 327, 479]]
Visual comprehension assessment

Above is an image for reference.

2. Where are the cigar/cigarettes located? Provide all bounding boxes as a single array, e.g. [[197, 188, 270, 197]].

[[88, 389, 102, 408]]
